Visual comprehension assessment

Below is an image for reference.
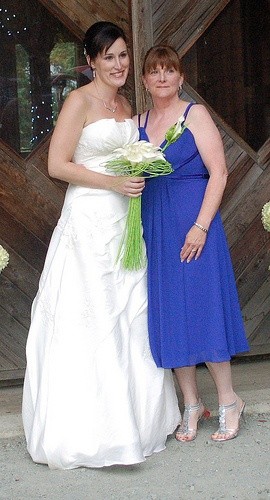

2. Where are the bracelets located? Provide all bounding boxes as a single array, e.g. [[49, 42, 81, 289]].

[[193, 221, 209, 233]]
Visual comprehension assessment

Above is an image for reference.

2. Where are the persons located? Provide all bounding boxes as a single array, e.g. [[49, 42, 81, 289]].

[[46, 20, 184, 452], [129, 45, 248, 442]]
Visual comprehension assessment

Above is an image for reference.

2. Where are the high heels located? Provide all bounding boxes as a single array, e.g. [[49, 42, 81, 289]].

[[211, 392, 246, 441], [176, 398, 206, 442]]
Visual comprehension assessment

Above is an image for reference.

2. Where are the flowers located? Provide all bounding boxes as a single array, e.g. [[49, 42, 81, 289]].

[[0, 245, 9, 273], [261, 201, 270, 232], [162, 116, 188, 153], [103, 140, 175, 269]]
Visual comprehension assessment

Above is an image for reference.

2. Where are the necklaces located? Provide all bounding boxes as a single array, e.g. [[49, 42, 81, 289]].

[[93, 79, 118, 112]]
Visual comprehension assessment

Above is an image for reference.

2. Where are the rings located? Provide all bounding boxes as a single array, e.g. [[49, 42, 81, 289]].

[[192, 249, 197, 254]]
